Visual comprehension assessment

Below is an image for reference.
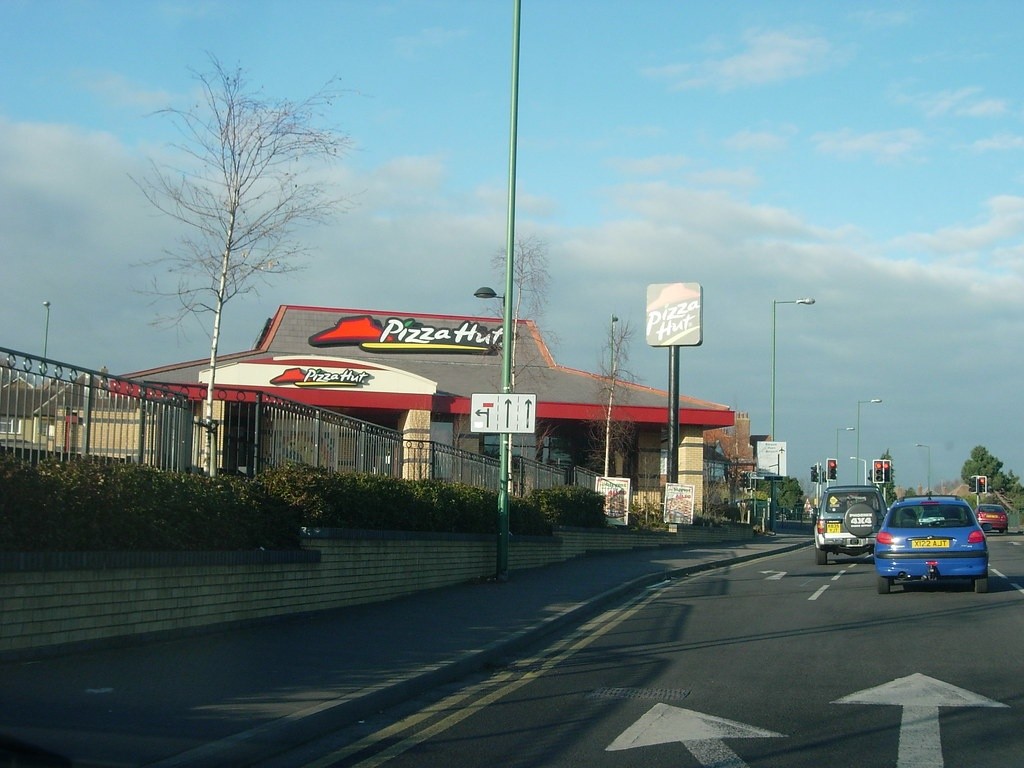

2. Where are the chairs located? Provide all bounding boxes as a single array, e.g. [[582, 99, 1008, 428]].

[[942, 518, 960, 527], [899, 517, 916, 526]]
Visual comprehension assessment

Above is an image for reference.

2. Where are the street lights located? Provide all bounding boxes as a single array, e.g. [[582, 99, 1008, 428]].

[[38, 301, 51, 435], [770, 299, 816, 530], [856, 398, 882, 484], [916, 444, 930, 495], [834, 428, 855, 485], [851, 457, 867, 486], [610, 312, 619, 380], [474, 286, 511, 580]]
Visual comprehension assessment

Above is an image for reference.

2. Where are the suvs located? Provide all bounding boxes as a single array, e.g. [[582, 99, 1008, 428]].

[[811, 485, 887, 565]]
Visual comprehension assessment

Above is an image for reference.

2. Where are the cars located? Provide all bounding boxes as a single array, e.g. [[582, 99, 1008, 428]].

[[974, 505, 1008, 535], [874, 495, 988, 593]]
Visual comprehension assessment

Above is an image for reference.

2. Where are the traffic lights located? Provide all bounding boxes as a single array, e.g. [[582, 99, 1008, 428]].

[[978, 476, 988, 495], [968, 475, 978, 494], [874, 459, 892, 484], [826, 458, 837, 481], [811, 463, 820, 484]]
